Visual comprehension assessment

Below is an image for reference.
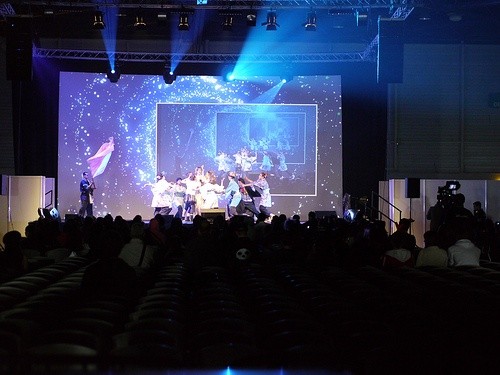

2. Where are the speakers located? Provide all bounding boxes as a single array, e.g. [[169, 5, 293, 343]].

[[404, 178, 420, 198], [201, 209, 225, 223], [308, 210, 337, 219]]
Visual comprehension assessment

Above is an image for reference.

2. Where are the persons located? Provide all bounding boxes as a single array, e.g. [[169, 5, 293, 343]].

[[0, 202, 495, 279], [145, 165, 276, 223], [79, 172, 96, 216]]
